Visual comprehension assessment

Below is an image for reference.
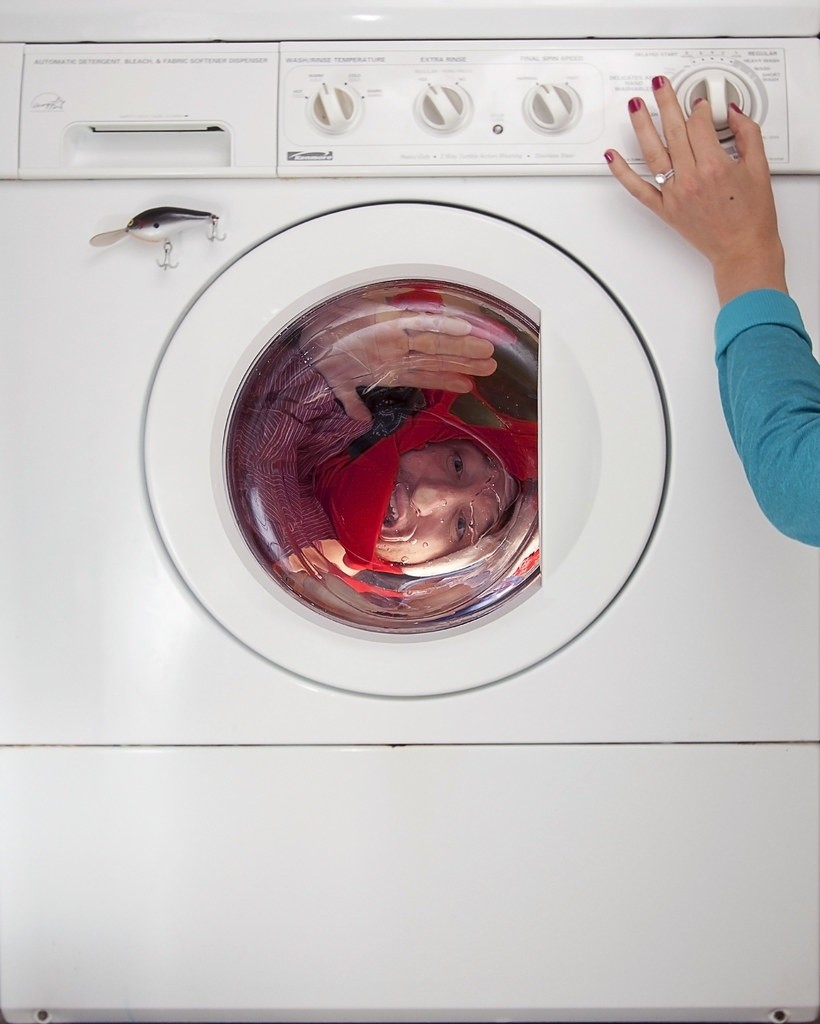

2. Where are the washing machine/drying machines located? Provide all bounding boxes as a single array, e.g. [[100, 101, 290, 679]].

[[0, 0, 820, 1024]]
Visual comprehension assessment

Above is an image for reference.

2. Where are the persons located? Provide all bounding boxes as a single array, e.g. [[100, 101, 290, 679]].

[[603, 75, 819, 550], [226, 282, 540, 626]]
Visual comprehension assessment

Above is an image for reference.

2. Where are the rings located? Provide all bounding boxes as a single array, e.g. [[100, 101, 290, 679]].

[[655, 169, 675, 184]]
